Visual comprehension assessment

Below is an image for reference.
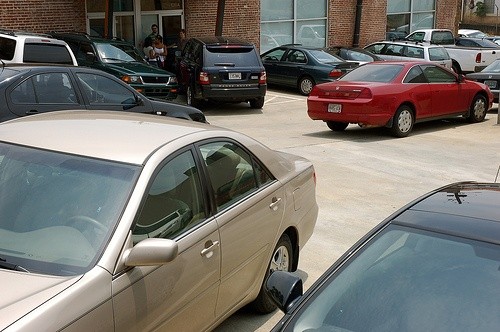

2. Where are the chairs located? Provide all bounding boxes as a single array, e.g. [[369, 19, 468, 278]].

[[138, 168, 190, 227], [289, 55, 296, 62]]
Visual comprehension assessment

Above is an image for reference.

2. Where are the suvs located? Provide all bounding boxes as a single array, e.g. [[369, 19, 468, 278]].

[[175, 37, 267, 112], [45, 30, 178, 101], [1, 28, 95, 92]]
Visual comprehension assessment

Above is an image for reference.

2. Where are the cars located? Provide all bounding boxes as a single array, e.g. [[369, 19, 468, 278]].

[[0, 108, 319, 332], [260, 24, 326, 52], [0, 64, 209, 124], [388, 14, 433, 35], [307, 62, 494, 139], [263, 181, 500, 332], [326, 28, 500, 104], [259, 43, 359, 96]]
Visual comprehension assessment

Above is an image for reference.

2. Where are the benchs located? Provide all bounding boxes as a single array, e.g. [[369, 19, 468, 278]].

[[206, 148, 255, 205]]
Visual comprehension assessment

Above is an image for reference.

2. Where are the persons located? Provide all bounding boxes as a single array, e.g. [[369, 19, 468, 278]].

[[178, 29, 188, 49], [143, 37, 158, 66], [154, 35, 167, 69], [149, 24, 158, 48]]
